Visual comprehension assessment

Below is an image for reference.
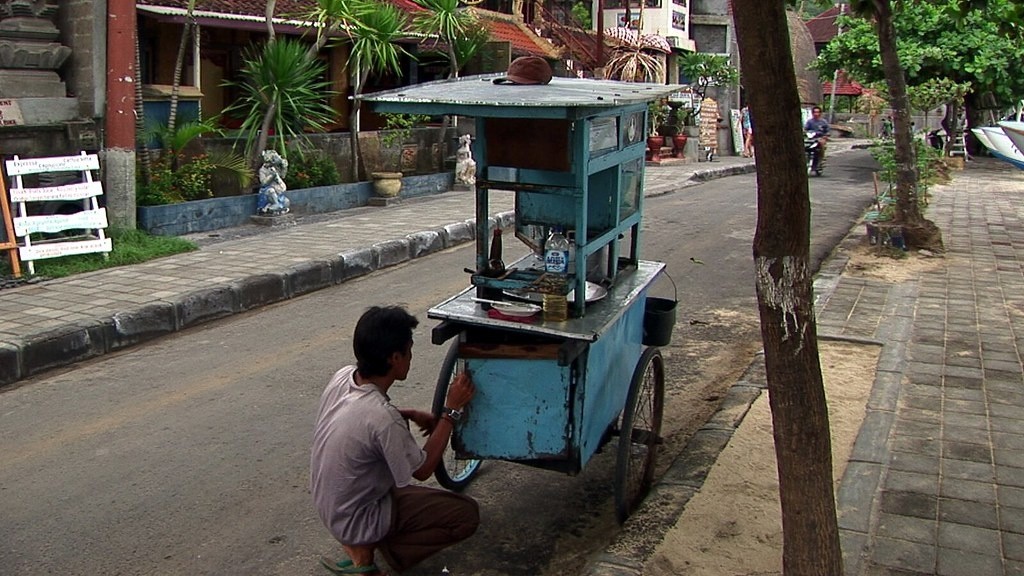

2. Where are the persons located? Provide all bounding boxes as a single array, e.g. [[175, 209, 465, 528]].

[[804, 106, 830, 175], [310, 305, 480, 576], [735, 106, 753, 157], [882, 116, 893, 139]]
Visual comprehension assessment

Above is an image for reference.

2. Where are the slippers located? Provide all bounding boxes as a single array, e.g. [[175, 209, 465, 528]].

[[320, 556, 390, 576]]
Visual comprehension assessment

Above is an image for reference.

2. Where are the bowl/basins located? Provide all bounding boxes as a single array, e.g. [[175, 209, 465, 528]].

[[502, 282, 610, 304]]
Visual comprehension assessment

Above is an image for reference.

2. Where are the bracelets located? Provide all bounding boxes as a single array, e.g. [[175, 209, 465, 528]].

[[440, 417, 454, 425]]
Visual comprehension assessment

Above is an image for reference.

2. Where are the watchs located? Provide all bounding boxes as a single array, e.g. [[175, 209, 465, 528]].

[[442, 407, 462, 421]]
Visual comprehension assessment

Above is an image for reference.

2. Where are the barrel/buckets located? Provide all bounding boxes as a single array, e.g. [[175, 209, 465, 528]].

[[642, 269, 681, 346]]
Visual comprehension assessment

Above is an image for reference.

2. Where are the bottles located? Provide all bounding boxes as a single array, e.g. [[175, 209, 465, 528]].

[[542, 224, 568, 322], [481, 230, 504, 310]]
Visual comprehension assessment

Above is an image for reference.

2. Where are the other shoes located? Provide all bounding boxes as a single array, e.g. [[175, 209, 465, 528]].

[[743, 152, 748, 158], [750, 152, 753, 157]]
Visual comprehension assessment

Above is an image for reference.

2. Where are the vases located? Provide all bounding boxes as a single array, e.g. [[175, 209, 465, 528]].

[[371, 172, 404, 197]]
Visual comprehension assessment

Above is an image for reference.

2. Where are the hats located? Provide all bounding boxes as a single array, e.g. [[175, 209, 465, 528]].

[[493, 56, 552, 85]]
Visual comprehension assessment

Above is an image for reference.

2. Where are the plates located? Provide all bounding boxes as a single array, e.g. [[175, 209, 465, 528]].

[[491, 302, 540, 316]]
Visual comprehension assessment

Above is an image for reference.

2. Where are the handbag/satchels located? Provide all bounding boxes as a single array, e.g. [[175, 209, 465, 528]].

[[748, 127, 753, 134]]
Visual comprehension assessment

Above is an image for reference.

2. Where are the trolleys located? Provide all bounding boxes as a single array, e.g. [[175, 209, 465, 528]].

[[350, 69, 693, 527]]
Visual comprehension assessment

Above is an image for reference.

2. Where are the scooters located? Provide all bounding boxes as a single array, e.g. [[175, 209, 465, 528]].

[[802, 129, 829, 178]]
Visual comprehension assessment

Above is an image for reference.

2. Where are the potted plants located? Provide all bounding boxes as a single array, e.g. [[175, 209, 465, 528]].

[[646, 102, 671, 154], [670, 110, 689, 160]]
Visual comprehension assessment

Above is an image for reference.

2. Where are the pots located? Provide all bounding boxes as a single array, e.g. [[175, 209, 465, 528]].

[[567, 232, 625, 278]]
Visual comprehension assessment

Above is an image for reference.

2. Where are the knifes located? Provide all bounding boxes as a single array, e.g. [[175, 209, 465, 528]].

[[469, 297, 541, 309]]
